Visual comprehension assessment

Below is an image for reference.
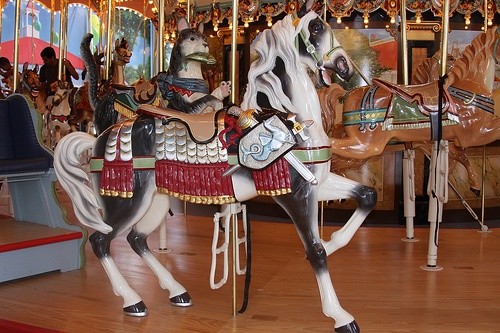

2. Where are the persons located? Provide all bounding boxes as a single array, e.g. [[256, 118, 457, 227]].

[[39, 47, 79, 94], [0, 57, 20, 82]]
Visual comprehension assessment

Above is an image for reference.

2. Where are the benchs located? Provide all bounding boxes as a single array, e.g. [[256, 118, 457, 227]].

[[0, 91, 88, 285]]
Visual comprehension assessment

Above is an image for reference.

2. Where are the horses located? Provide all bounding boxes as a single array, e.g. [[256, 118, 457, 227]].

[[0, 1, 499, 333]]
